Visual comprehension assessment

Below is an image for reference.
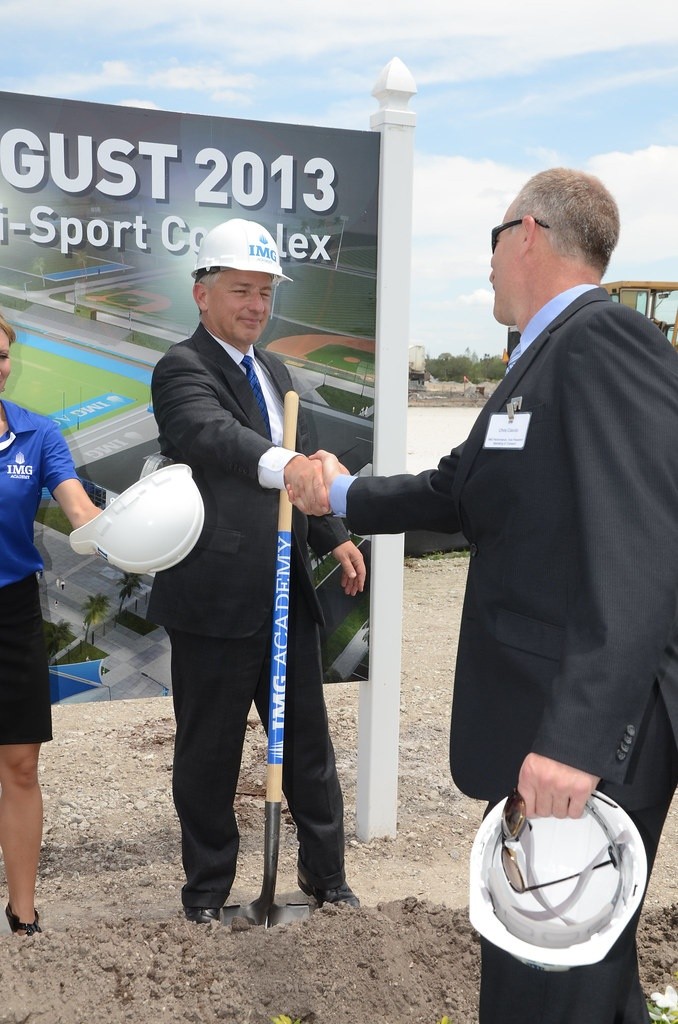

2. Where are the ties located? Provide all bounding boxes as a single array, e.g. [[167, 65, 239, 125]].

[[505, 342, 521, 377], [241, 356, 272, 442]]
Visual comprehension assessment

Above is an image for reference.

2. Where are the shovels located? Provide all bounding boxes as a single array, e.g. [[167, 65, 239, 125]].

[[219, 388, 313, 931]]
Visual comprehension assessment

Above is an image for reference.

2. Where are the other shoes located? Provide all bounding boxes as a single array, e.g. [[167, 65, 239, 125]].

[[5, 902, 42, 937]]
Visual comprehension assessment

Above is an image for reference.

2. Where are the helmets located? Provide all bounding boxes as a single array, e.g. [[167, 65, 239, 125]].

[[191, 219, 293, 286], [469, 788, 647, 973], [68, 463, 205, 575]]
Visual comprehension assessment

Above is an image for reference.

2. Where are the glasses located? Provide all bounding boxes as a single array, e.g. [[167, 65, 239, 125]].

[[491, 217, 550, 254], [501, 785, 618, 894]]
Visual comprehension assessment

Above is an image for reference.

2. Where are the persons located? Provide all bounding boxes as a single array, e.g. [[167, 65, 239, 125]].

[[0, 314, 105, 935], [314, 164, 677, 1024], [153, 217, 374, 925]]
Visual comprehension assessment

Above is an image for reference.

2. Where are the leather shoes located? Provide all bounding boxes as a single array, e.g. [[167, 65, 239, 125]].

[[184, 907, 220, 924], [298, 876, 360, 910]]
